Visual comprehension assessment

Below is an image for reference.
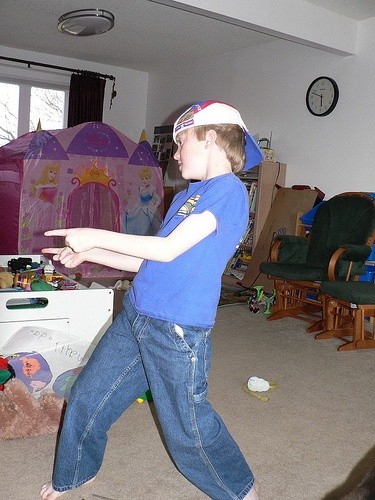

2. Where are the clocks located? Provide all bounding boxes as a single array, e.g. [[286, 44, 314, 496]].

[[306, 76, 339, 116]]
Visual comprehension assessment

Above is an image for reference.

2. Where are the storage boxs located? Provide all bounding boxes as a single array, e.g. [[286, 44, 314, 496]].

[[244, 186, 319, 293]]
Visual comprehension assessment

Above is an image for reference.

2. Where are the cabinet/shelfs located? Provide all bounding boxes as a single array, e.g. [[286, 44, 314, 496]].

[[0, 253, 114, 403], [221, 162, 286, 286]]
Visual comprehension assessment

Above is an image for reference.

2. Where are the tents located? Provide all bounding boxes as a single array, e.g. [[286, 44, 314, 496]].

[[0, 121, 164, 279]]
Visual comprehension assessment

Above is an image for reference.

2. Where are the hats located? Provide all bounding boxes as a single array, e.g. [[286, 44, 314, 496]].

[[173, 99, 265, 174]]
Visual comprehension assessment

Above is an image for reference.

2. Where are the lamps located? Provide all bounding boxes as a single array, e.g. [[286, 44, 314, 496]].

[[57, 9, 115, 36]]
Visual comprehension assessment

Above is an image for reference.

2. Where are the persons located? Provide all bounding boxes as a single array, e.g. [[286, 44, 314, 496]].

[[40, 100, 265, 500]]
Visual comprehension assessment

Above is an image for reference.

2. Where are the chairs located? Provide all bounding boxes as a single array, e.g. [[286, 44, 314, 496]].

[[262, 192, 375, 351]]
